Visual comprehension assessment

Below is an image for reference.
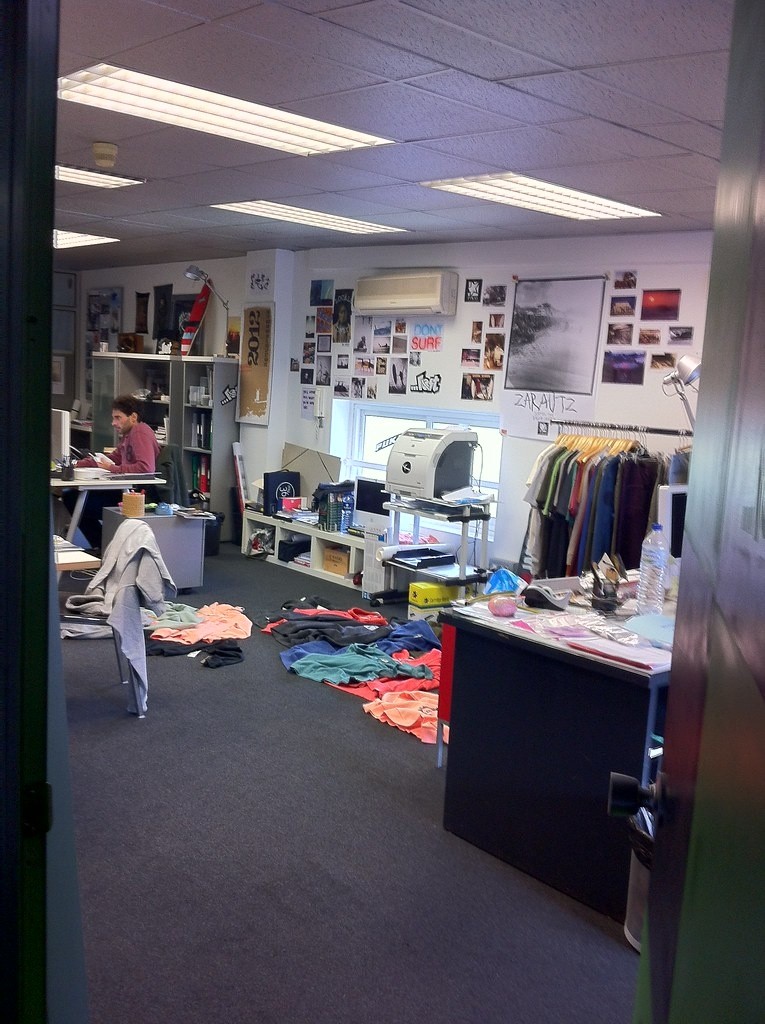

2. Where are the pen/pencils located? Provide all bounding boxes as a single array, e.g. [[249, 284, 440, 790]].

[[55, 455, 76, 468], [126, 488, 146, 496]]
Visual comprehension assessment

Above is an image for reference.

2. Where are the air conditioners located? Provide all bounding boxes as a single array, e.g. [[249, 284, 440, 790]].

[[354, 271, 459, 318]]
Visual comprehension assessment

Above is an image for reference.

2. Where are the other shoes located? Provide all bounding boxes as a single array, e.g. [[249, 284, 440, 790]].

[[83, 546, 101, 557]]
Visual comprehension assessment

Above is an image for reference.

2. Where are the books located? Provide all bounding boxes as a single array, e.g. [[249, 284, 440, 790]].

[[154, 425, 167, 440]]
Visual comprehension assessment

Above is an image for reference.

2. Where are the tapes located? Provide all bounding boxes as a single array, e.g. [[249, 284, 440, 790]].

[[542, 586, 554, 597]]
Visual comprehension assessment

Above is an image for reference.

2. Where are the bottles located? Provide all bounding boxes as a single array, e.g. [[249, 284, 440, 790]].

[[340, 491, 355, 533], [637, 524, 668, 617]]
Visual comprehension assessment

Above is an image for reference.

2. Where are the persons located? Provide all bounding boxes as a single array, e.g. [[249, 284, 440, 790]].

[[62, 393, 162, 558], [301, 284, 505, 399]]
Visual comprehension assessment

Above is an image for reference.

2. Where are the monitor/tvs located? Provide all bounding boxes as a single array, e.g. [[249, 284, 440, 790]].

[[660, 484, 688, 574], [51, 409, 71, 477]]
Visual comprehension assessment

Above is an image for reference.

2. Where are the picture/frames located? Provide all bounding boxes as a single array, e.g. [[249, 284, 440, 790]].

[[51, 354, 67, 395], [317, 335, 332, 353]]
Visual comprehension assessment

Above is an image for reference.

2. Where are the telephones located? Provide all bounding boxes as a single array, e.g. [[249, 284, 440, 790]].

[[313, 386, 327, 418]]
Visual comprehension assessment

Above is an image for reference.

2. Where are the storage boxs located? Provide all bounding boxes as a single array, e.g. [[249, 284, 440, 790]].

[[322, 546, 349, 576], [407, 582, 473, 623]]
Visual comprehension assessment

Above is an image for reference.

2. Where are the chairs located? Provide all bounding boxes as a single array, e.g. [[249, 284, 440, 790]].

[[55, 519, 177, 719]]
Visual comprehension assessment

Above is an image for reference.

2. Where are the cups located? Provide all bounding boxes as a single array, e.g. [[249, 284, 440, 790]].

[[189, 386, 205, 405], [62, 466, 74, 481], [201, 395, 210, 405], [122, 492, 145, 517], [100, 342, 109, 352]]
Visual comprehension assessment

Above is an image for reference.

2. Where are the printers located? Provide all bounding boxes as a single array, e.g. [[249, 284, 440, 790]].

[[385, 428, 478, 500]]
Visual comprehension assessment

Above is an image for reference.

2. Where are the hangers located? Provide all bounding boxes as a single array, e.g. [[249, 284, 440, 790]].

[[554, 420, 664, 466], [674, 429, 692, 455]]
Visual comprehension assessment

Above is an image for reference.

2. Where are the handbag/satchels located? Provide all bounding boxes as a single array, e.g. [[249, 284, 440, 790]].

[[264, 469, 300, 516]]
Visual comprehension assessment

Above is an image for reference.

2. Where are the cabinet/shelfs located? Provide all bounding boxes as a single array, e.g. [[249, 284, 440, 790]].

[[91, 351, 240, 541], [102, 507, 206, 589], [369, 490, 491, 624], [241, 510, 432, 600], [71, 421, 92, 433]]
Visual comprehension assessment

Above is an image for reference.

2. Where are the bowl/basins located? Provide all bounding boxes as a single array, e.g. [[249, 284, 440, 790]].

[[118, 502, 123, 511]]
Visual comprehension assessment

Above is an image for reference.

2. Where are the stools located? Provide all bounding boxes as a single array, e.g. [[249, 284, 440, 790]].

[[278, 540, 311, 563]]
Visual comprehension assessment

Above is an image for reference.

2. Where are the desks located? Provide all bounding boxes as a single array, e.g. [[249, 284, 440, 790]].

[[50, 472, 167, 586], [435, 565, 681, 926], [54, 533, 101, 572]]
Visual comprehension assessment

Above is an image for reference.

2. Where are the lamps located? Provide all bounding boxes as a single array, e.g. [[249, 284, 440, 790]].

[[92, 142, 118, 167], [662, 354, 702, 431], [184, 265, 231, 312]]
